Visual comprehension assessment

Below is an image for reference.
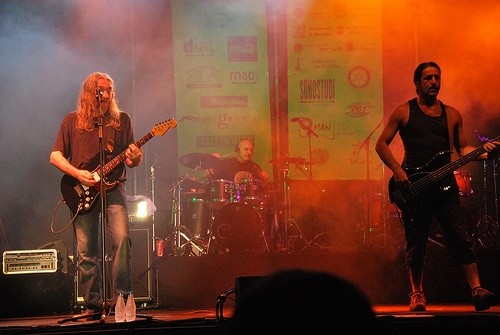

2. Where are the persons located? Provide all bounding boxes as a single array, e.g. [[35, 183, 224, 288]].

[[203, 139, 269, 184], [49, 72, 145, 321], [375, 62, 500, 312]]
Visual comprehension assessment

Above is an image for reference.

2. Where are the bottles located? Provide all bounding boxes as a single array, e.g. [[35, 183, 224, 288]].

[[115, 292, 126, 323], [125, 290, 136, 322]]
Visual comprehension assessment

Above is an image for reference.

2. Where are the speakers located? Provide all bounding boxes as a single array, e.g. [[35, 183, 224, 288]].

[[234, 275, 266, 308]]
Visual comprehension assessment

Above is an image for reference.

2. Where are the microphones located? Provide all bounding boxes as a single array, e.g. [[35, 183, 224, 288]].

[[473, 129, 481, 141], [95, 82, 102, 100]]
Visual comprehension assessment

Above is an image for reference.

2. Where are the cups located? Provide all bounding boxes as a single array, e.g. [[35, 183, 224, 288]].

[[156, 239, 164, 257]]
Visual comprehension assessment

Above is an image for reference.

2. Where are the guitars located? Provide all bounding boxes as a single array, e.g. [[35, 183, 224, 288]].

[[387, 134, 500, 216], [61, 117, 178, 215]]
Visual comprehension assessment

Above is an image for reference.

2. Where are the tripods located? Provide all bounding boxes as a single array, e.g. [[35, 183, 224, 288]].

[[57, 96, 153, 324], [159, 164, 208, 256]]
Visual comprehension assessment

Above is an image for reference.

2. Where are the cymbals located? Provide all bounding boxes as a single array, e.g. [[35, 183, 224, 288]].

[[178, 179, 208, 190], [267, 156, 321, 166], [179, 152, 220, 171]]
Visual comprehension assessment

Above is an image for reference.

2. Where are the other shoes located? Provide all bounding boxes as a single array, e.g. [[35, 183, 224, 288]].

[[71, 308, 101, 321], [111, 299, 127, 313]]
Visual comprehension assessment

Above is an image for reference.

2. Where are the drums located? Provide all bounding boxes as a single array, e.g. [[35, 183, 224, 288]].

[[169, 178, 283, 257], [453, 170, 475, 200], [354, 191, 388, 233]]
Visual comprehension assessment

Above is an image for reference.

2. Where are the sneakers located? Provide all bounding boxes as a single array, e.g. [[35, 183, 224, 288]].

[[409, 291, 427, 311], [472, 288, 494, 311]]
